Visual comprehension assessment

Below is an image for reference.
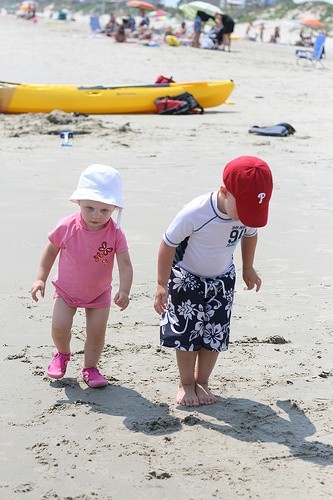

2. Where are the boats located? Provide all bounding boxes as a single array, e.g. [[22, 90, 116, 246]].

[[0, 78, 235, 115]]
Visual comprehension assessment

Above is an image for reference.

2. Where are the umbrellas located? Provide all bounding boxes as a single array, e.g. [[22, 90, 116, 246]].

[[7, 1, 326, 29]]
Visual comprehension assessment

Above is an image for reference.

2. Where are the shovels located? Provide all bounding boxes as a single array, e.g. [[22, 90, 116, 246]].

[[59, 131, 73, 146]]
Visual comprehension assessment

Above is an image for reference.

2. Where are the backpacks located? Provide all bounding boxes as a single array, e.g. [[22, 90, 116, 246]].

[[154, 92, 205, 114]]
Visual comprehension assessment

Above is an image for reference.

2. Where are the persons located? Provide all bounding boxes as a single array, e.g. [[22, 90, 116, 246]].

[[0, 0, 324, 51], [30, 164, 133, 387], [154, 156, 273, 407]]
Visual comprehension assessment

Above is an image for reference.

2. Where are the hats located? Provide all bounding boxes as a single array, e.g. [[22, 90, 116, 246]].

[[69, 163, 127, 230], [222, 156, 273, 229]]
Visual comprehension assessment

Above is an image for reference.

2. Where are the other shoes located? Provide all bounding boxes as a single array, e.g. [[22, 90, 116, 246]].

[[82, 367, 108, 388], [46, 352, 71, 378]]
